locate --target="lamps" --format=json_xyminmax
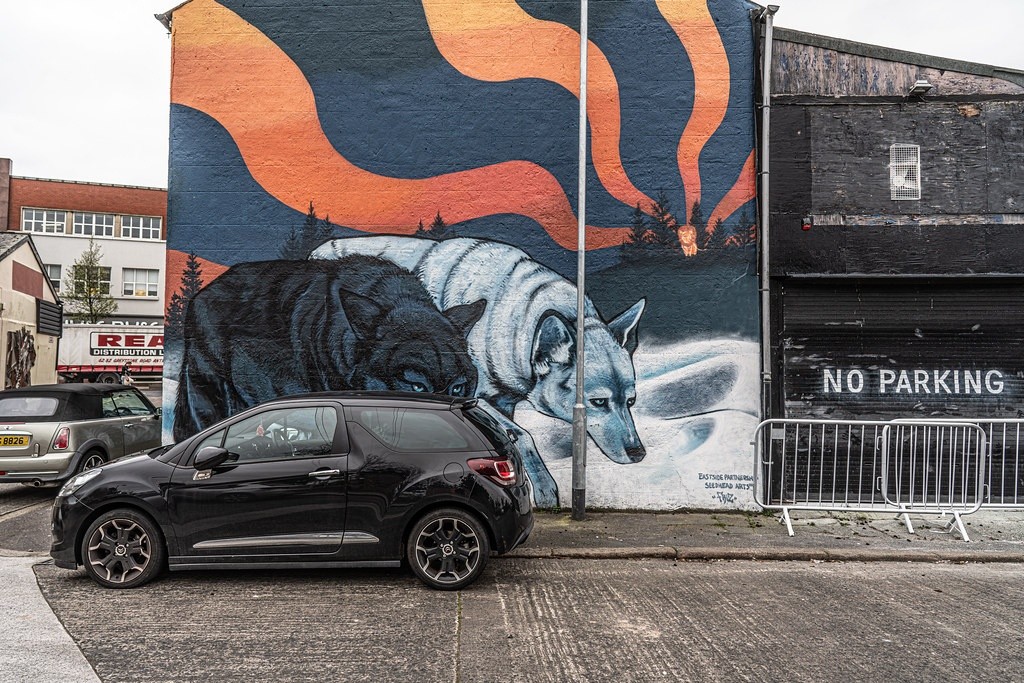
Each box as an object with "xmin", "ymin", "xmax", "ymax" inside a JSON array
[{"xmin": 909, "ymin": 79, "xmax": 935, "ymax": 95}]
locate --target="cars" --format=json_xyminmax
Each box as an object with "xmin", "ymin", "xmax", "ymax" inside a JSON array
[{"xmin": 0, "ymin": 382, "xmax": 163, "ymax": 500}]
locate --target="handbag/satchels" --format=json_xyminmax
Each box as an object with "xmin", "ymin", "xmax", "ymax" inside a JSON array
[{"xmin": 121, "ymin": 373, "xmax": 133, "ymax": 386}]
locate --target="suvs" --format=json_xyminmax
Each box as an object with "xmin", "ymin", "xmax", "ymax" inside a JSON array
[{"xmin": 49, "ymin": 389, "xmax": 539, "ymax": 590}]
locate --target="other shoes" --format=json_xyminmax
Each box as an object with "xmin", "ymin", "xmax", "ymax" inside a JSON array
[
  {"xmin": 118, "ymin": 395, "xmax": 123, "ymax": 397},
  {"xmin": 126, "ymin": 394, "xmax": 130, "ymax": 396}
]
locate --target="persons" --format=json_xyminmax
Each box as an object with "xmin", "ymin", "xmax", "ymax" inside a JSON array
[{"xmin": 119, "ymin": 362, "xmax": 134, "ymax": 397}]
